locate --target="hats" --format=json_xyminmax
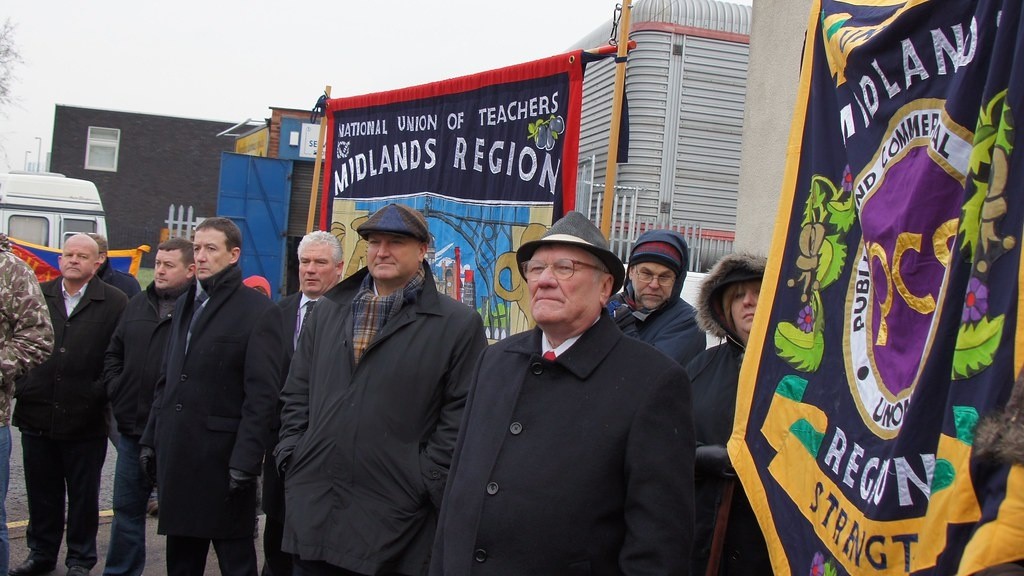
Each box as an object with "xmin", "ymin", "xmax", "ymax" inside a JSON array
[
  {"xmin": 629, "ymin": 241, "xmax": 683, "ymax": 277},
  {"xmin": 243, "ymin": 275, "xmax": 271, "ymax": 300},
  {"xmin": 516, "ymin": 211, "xmax": 625, "ymax": 298},
  {"xmin": 357, "ymin": 202, "xmax": 431, "ymax": 244}
]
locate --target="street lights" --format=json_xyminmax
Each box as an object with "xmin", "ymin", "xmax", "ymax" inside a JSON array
[
  {"xmin": 25, "ymin": 151, "xmax": 32, "ymax": 170},
  {"xmin": 35, "ymin": 138, "xmax": 41, "ymax": 173}
]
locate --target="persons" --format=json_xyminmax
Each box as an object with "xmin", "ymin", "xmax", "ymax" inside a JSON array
[
  {"xmin": 429, "ymin": 212, "xmax": 772, "ymax": 576},
  {"xmin": 0, "ymin": 217, "xmax": 344, "ymax": 576},
  {"xmin": 281, "ymin": 204, "xmax": 487, "ymax": 576},
  {"xmin": 940, "ymin": 365, "xmax": 1024, "ymax": 576}
]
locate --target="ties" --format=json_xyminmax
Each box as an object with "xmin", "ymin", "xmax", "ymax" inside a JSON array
[{"xmin": 297, "ymin": 300, "xmax": 315, "ymax": 337}]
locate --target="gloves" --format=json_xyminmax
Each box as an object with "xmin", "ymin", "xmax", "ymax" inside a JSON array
[
  {"xmin": 228, "ymin": 476, "xmax": 259, "ymax": 494},
  {"xmin": 696, "ymin": 442, "xmax": 739, "ymax": 478},
  {"xmin": 139, "ymin": 445, "xmax": 157, "ymax": 487}
]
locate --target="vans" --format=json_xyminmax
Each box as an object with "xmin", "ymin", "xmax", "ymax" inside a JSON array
[{"xmin": 0, "ymin": 171, "xmax": 109, "ymax": 251}]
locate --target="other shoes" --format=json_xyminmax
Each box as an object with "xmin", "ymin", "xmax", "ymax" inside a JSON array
[{"xmin": 147, "ymin": 492, "xmax": 159, "ymax": 515}]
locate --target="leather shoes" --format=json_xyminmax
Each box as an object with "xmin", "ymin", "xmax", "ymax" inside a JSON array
[
  {"xmin": 9, "ymin": 559, "xmax": 56, "ymax": 576},
  {"xmin": 66, "ymin": 566, "xmax": 89, "ymax": 576}
]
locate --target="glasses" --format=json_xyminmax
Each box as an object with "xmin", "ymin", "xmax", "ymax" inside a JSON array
[
  {"xmin": 634, "ymin": 264, "xmax": 675, "ymax": 286},
  {"xmin": 520, "ymin": 258, "xmax": 608, "ymax": 281}
]
